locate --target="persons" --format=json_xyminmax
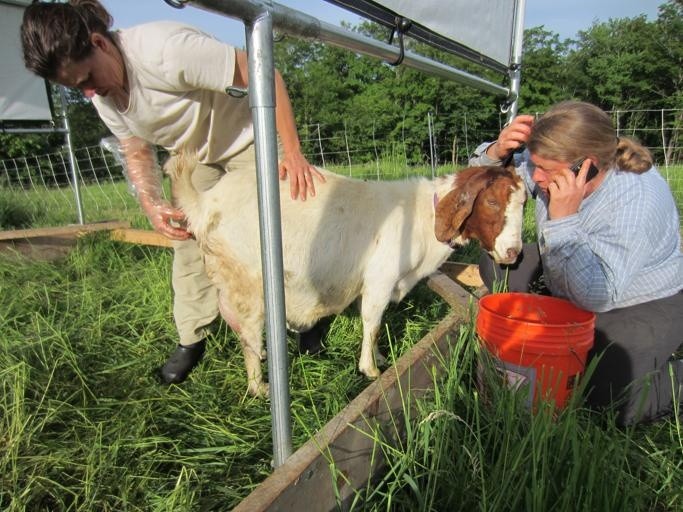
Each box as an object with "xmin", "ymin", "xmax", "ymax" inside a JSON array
[
  {"xmin": 21, "ymin": 0, "xmax": 326, "ymax": 381},
  {"xmin": 467, "ymin": 101, "xmax": 682, "ymax": 426}
]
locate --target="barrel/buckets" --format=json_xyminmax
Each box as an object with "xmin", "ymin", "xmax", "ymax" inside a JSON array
[{"xmin": 476, "ymin": 292, "xmax": 596, "ymax": 417}]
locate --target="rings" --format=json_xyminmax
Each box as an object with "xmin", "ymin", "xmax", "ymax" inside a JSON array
[{"xmin": 304, "ymin": 172, "xmax": 311, "ymax": 177}]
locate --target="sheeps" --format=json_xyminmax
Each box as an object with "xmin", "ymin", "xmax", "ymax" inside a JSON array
[{"xmin": 167, "ymin": 145, "xmax": 529, "ymax": 401}]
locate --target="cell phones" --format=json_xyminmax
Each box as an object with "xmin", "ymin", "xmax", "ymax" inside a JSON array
[{"xmin": 545, "ymin": 158, "xmax": 599, "ymax": 196}]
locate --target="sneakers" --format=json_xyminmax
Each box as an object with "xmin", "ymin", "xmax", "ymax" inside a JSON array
[
  {"xmin": 299, "ymin": 327, "xmax": 322, "ymax": 355},
  {"xmin": 162, "ymin": 345, "xmax": 202, "ymax": 382}
]
